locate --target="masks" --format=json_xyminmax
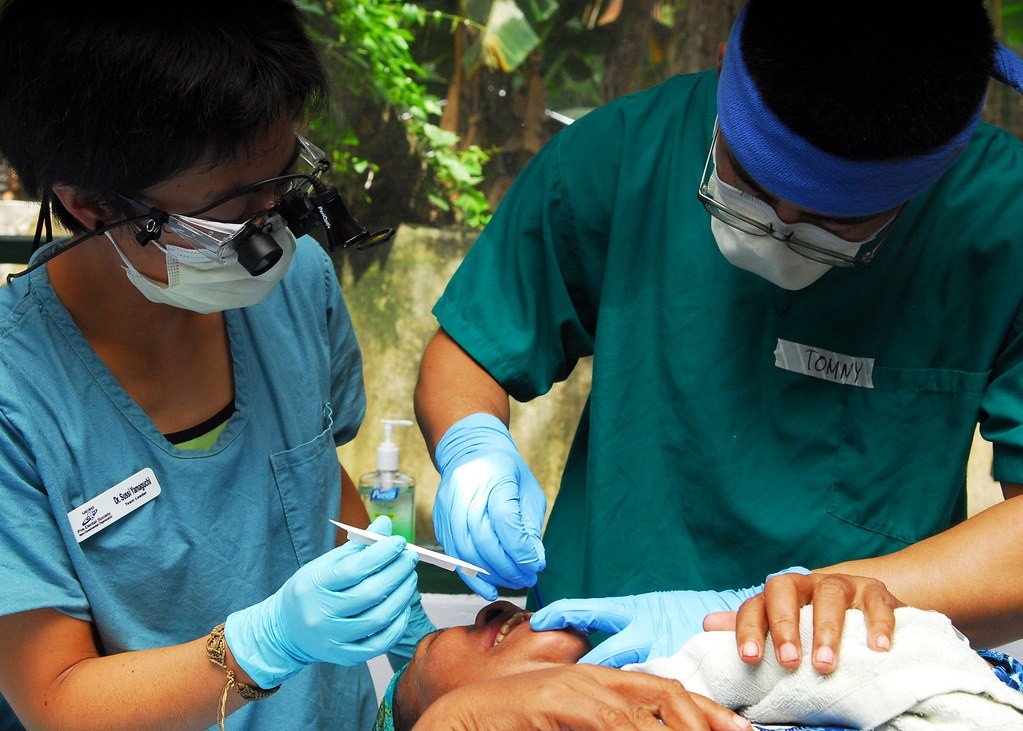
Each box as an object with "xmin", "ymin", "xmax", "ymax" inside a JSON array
[
  {"xmin": 95, "ymin": 201, "xmax": 298, "ymax": 315},
  {"xmin": 708, "ymin": 117, "xmax": 897, "ymax": 292}
]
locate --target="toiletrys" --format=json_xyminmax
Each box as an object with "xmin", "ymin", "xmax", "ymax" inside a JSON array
[{"xmin": 359, "ymin": 418, "xmax": 417, "ymax": 545}]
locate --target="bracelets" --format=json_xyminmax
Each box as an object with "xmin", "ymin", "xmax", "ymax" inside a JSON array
[{"xmin": 206, "ymin": 624, "xmax": 282, "ymax": 730}]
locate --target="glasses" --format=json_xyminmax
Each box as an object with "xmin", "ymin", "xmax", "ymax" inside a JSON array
[
  {"xmin": 118, "ymin": 129, "xmax": 334, "ymax": 264},
  {"xmin": 697, "ymin": 117, "xmax": 912, "ymax": 271}
]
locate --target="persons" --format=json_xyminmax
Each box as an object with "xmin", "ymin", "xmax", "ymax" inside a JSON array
[
  {"xmin": 373, "ymin": 601, "xmax": 1022, "ymax": 731},
  {"xmin": 0, "ymin": 0, "xmax": 435, "ymax": 731},
  {"xmin": 414, "ymin": 0, "xmax": 1022, "ymax": 673}
]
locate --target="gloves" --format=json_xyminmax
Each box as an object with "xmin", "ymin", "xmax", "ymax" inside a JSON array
[
  {"xmin": 433, "ymin": 414, "xmax": 548, "ymax": 600},
  {"xmin": 528, "ymin": 565, "xmax": 814, "ymax": 668},
  {"xmin": 220, "ymin": 514, "xmax": 417, "ymax": 688},
  {"xmin": 380, "ymin": 588, "xmax": 436, "ymax": 675}
]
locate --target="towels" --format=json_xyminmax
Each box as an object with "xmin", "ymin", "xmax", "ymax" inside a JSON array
[{"xmin": 620, "ymin": 605, "xmax": 1022, "ymax": 731}]
